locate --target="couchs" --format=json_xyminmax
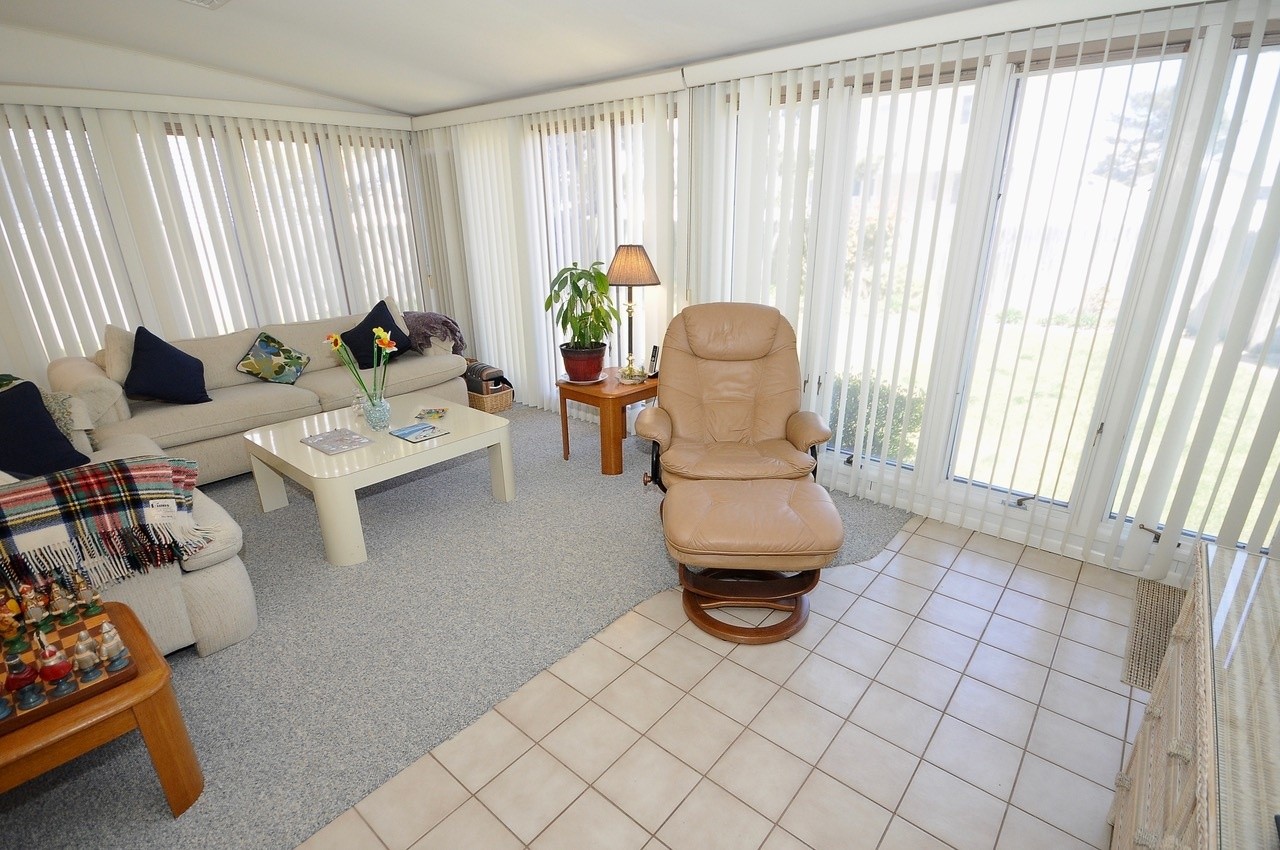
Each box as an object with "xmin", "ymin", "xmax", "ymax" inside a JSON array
[
  {"xmin": 44, "ymin": 312, "xmax": 467, "ymax": 489},
  {"xmin": 0, "ymin": 389, "xmax": 257, "ymax": 656}
]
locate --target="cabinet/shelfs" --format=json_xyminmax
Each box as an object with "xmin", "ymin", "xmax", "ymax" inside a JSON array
[{"xmin": 1112, "ymin": 543, "xmax": 1280, "ymax": 850}]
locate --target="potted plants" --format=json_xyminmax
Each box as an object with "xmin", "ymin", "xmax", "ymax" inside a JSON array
[{"xmin": 543, "ymin": 260, "xmax": 622, "ymax": 386}]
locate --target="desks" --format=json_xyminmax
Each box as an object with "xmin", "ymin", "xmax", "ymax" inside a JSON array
[
  {"xmin": 1, "ymin": 602, "xmax": 207, "ymax": 818},
  {"xmin": 556, "ymin": 367, "xmax": 659, "ymax": 475}
]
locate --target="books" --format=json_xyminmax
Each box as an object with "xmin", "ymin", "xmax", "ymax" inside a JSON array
[{"xmin": 300, "ymin": 427, "xmax": 377, "ymax": 456}]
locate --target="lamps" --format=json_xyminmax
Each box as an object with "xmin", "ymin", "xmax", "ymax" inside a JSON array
[{"xmin": 607, "ymin": 245, "xmax": 662, "ymax": 387}]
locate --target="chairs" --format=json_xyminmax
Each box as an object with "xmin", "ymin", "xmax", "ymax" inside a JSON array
[{"xmin": 635, "ymin": 304, "xmax": 844, "ymax": 647}]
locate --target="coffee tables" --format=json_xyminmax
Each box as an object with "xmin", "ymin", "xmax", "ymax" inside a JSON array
[{"xmin": 242, "ymin": 391, "xmax": 519, "ymax": 566}]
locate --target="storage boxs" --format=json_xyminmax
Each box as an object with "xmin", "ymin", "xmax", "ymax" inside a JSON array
[{"xmin": 463, "ymin": 362, "xmax": 504, "ymax": 394}]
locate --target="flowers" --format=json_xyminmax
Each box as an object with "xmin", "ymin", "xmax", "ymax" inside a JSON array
[{"xmin": 324, "ymin": 327, "xmax": 398, "ymax": 404}]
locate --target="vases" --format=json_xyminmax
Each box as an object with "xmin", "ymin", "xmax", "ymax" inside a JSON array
[{"xmin": 364, "ymin": 392, "xmax": 390, "ymax": 432}]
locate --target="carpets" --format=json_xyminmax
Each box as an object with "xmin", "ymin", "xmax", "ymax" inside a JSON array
[
  {"xmin": 0, "ymin": 399, "xmax": 921, "ymax": 849},
  {"xmin": 1123, "ymin": 578, "xmax": 1188, "ymax": 693}
]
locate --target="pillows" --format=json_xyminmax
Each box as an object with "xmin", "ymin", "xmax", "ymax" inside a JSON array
[
  {"xmin": 127, "ymin": 327, "xmax": 212, "ymax": 402},
  {"xmin": 101, "ymin": 323, "xmax": 135, "ymax": 384},
  {"xmin": 341, "ymin": 301, "xmax": 412, "ymax": 368},
  {"xmin": 385, "ymin": 296, "xmax": 410, "ymax": 336},
  {"xmin": 238, "ymin": 331, "xmax": 311, "ymax": 384}
]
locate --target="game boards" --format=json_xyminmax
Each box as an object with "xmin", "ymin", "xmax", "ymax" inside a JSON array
[{"xmin": 0, "ymin": 596, "xmax": 138, "ymax": 737}]
